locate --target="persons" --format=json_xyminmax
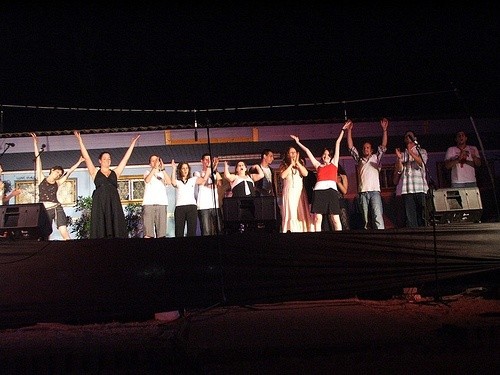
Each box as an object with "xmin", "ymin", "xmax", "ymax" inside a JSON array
[
  {"xmin": 74, "ymin": 130, "xmax": 140, "ymax": 239},
  {"xmin": 0, "ymin": 165, "xmax": 21, "ymax": 205},
  {"xmin": 444, "ymin": 130, "xmax": 481, "ymax": 188},
  {"xmin": 142, "ymin": 118, "xmax": 437, "ymax": 239},
  {"xmin": 29, "ymin": 133, "xmax": 85, "ymax": 240}
]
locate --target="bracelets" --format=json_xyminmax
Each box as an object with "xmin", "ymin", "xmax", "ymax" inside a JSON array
[
  {"xmin": 383, "ymin": 129, "xmax": 387, "ymax": 132},
  {"xmin": 341, "ymin": 129, "xmax": 345, "ymax": 131},
  {"xmin": 161, "ymin": 168, "xmax": 165, "ymax": 171},
  {"xmin": 457, "ymin": 158, "xmax": 460, "ymax": 162}
]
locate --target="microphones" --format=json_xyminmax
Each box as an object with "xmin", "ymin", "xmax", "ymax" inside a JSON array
[
  {"xmin": 194, "ymin": 120, "xmax": 198, "ymax": 141},
  {"xmin": 40, "ymin": 144, "xmax": 46, "ymax": 152},
  {"xmin": 408, "ymin": 135, "xmax": 421, "ymax": 149},
  {"xmin": 6, "ymin": 143, "xmax": 15, "ymax": 147}
]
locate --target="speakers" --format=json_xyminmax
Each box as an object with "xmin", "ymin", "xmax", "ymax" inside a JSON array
[
  {"xmin": 425, "ymin": 187, "xmax": 483, "ymax": 226},
  {"xmin": 222, "ymin": 195, "xmax": 282, "ymax": 234},
  {"xmin": 0, "ymin": 203, "xmax": 52, "ymax": 241}
]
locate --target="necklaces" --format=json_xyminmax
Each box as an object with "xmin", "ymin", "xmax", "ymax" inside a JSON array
[{"xmin": 291, "ymin": 167, "xmax": 297, "ymax": 175}]
